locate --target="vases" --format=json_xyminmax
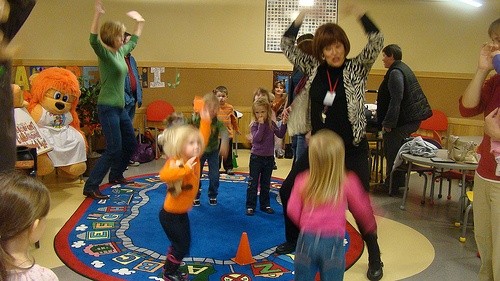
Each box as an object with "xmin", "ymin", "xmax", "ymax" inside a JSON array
[{"xmin": 85, "ymin": 151, "xmax": 102, "ymax": 177}]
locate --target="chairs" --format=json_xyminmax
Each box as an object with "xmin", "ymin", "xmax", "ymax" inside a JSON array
[
  {"xmin": 136, "ymin": 100, "xmax": 175, "ymax": 160},
  {"xmin": 388, "ymin": 110, "xmax": 475, "ymax": 242}
]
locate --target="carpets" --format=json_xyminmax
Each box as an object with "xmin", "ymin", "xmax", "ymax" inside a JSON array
[{"xmin": 54, "ymin": 169, "xmax": 365, "ymax": 281}]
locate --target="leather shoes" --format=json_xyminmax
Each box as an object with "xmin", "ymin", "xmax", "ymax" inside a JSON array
[
  {"xmin": 84, "ymin": 189, "xmax": 108, "ymax": 199},
  {"xmin": 276, "ymin": 242, "xmax": 296, "ymax": 254},
  {"xmin": 109, "ymin": 177, "xmax": 134, "ymax": 184},
  {"xmin": 363, "ymin": 259, "xmax": 383, "ymax": 281}
]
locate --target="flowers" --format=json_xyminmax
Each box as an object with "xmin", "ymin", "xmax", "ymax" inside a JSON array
[{"xmin": 75, "ymin": 76, "xmax": 103, "ymax": 138}]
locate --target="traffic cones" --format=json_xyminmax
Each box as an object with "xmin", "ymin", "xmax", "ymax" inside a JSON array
[{"xmin": 230, "ymin": 232, "xmax": 257, "ymax": 265}]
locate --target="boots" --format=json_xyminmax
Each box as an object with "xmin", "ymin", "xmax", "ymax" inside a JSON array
[
  {"xmin": 162, "ymin": 246, "xmax": 173, "ymax": 272},
  {"xmin": 163, "ymin": 253, "xmax": 189, "ymax": 281}
]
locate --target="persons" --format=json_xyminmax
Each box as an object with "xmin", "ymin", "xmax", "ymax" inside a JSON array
[
  {"xmin": 122, "ymin": 31, "xmax": 140, "ymax": 169},
  {"xmin": 159, "ymin": 109, "xmax": 211, "ymax": 281},
  {"xmin": 246, "ymin": 80, "xmax": 288, "ymax": 215},
  {"xmin": 376, "ymin": 43, "xmax": 433, "ymax": 193},
  {"xmin": 82, "ymin": 0, "xmax": 145, "ymax": 199},
  {"xmin": 184, "ymin": 86, "xmax": 240, "ymax": 208},
  {"xmin": 459, "ymin": 16, "xmax": 500, "ymax": 281},
  {"xmin": 286, "ymin": 129, "xmax": 376, "ymax": 281},
  {"xmin": 276, "ymin": 9, "xmax": 385, "ymax": 281},
  {"xmin": 289, "ymin": 31, "xmax": 317, "ymax": 162},
  {"xmin": 0, "ymin": 170, "xmax": 59, "ymax": 281}
]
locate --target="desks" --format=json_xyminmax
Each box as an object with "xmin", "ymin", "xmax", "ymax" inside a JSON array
[{"xmin": 400, "ymin": 136, "xmax": 484, "ymax": 225}]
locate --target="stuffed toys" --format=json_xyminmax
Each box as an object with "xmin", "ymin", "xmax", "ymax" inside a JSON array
[{"xmin": 23, "ymin": 65, "xmax": 88, "ymax": 176}]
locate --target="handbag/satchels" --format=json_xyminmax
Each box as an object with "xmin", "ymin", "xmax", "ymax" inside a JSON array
[
  {"xmin": 284, "ymin": 143, "xmax": 294, "ymax": 159},
  {"xmin": 132, "ymin": 131, "xmax": 160, "ymax": 163},
  {"xmin": 448, "ymin": 135, "xmax": 479, "ymax": 165}
]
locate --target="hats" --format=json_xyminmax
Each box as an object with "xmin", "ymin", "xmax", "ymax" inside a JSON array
[{"xmin": 297, "ymin": 33, "xmax": 314, "ymax": 46}]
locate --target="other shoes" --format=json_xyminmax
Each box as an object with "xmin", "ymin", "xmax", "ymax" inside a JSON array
[
  {"xmin": 209, "ymin": 198, "xmax": 217, "ymax": 205},
  {"xmin": 192, "ymin": 200, "xmax": 200, "ymax": 206},
  {"xmin": 374, "ymin": 184, "xmax": 398, "ymax": 194},
  {"xmin": 247, "ymin": 208, "xmax": 254, "ymax": 215},
  {"xmin": 227, "ymin": 169, "xmax": 235, "ymax": 174},
  {"xmin": 264, "ymin": 207, "xmax": 273, "ymax": 214}
]
